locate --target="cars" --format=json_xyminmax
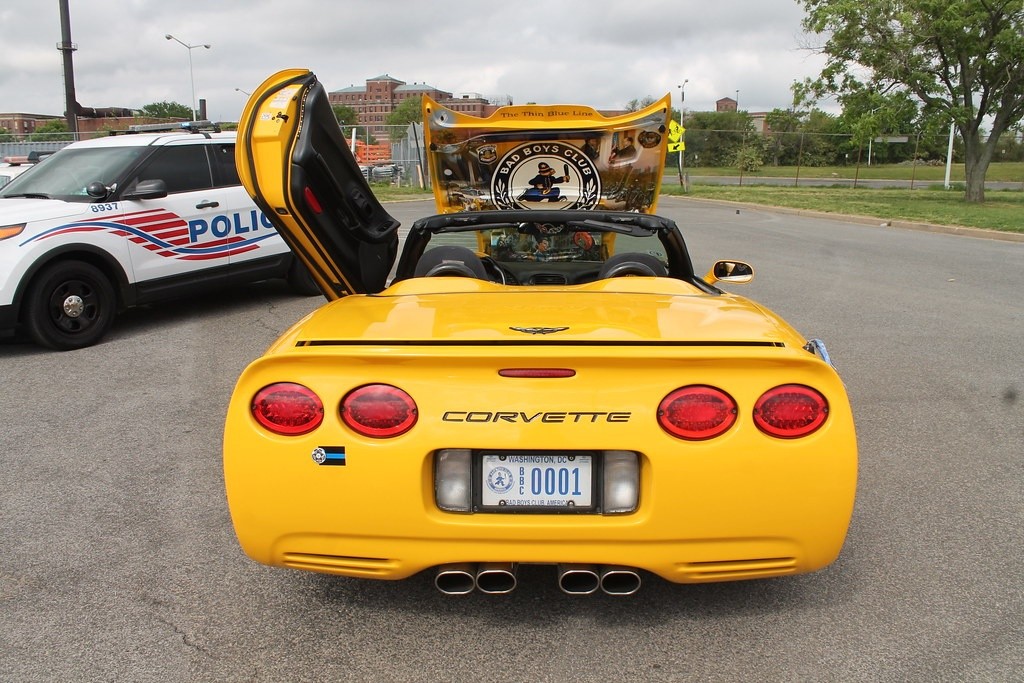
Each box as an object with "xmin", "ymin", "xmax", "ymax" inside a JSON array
[{"xmin": 0, "ymin": 120, "xmax": 325, "ymax": 351}]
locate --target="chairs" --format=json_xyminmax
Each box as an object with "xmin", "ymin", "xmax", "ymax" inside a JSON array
[
  {"xmin": 596, "ymin": 252, "xmax": 668, "ymax": 281},
  {"xmin": 413, "ymin": 245, "xmax": 489, "ymax": 282}
]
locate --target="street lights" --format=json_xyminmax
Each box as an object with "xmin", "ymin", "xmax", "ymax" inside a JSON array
[
  {"xmin": 165, "ymin": 33, "xmax": 211, "ymax": 120},
  {"xmin": 677, "ymin": 78, "xmax": 689, "ymax": 177}
]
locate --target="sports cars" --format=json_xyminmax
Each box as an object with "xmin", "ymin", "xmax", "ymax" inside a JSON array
[{"xmin": 222, "ymin": 68, "xmax": 861, "ymax": 596}]
